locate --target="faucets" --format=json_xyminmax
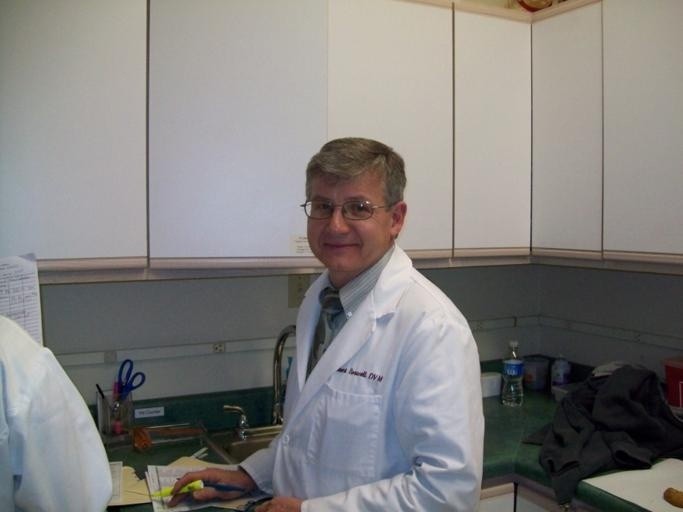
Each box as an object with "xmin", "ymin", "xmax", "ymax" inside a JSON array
[{"xmin": 271, "ymin": 325, "xmax": 296, "ymax": 426}]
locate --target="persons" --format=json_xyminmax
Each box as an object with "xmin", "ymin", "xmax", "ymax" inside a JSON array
[
  {"xmin": 1, "ymin": 313, "xmax": 115, "ymax": 512},
  {"xmin": 166, "ymin": 137, "xmax": 486, "ymax": 512}
]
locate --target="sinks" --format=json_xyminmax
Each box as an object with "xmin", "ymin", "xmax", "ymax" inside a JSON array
[{"xmin": 195, "ymin": 425, "xmax": 283, "ymax": 465}]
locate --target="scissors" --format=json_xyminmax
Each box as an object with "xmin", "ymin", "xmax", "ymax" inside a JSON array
[{"xmin": 111, "ymin": 359, "xmax": 145, "ymax": 414}]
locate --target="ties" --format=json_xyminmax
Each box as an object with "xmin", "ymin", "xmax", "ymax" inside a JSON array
[{"xmin": 306, "ymin": 287, "xmax": 342, "ymax": 386}]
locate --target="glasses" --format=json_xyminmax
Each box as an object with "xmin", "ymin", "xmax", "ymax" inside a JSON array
[{"xmin": 300, "ymin": 199, "xmax": 393, "ymax": 220}]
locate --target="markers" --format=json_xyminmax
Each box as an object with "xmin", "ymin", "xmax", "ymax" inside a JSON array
[{"xmin": 149, "ymin": 480, "xmax": 204, "ymax": 498}]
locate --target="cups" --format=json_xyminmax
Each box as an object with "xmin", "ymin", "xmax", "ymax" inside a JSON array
[
  {"xmin": 525, "ymin": 357, "xmax": 550, "ymax": 390},
  {"xmin": 94, "ymin": 389, "xmax": 134, "ymax": 444}
]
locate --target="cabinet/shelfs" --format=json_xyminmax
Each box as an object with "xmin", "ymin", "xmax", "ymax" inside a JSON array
[
  {"xmin": 476, "ymin": 480, "xmax": 516, "ymax": 511},
  {"xmin": 518, "ymin": 485, "xmax": 580, "ymax": 512},
  {"xmin": 533, "ymin": 1, "xmax": 683, "ymax": 275},
  {"xmin": 328, "ymin": 1, "xmax": 533, "ymax": 269},
  {"xmin": 1, "ymin": 2, "xmax": 326, "ymax": 273}
]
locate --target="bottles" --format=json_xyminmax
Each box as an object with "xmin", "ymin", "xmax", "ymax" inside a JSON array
[{"xmin": 500, "ymin": 340, "xmax": 525, "ymax": 408}]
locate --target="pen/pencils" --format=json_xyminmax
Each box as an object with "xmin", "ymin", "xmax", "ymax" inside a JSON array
[
  {"xmin": 96, "ymin": 382, "xmax": 123, "ymax": 435},
  {"xmin": 176, "ymin": 478, "xmax": 247, "ymax": 492}
]
